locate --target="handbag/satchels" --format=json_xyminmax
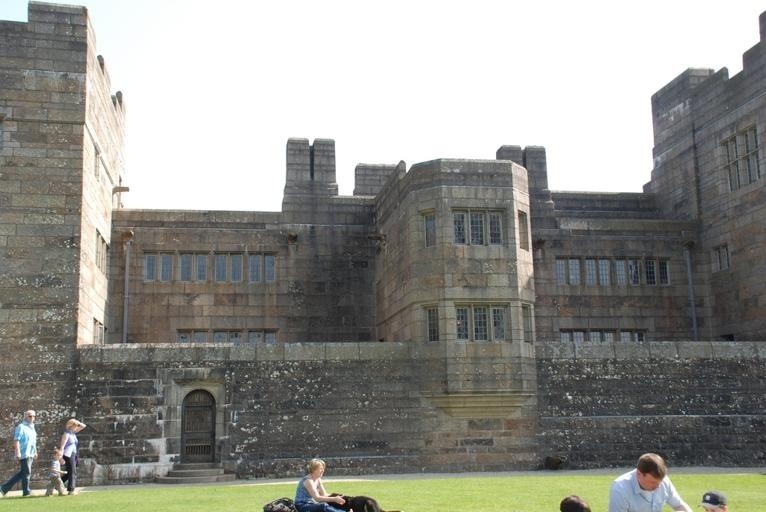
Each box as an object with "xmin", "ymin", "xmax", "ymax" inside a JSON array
[
  {"xmin": 75, "ymin": 454, "xmax": 79, "ymax": 465},
  {"xmin": 264, "ymin": 497, "xmax": 295, "ymax": 512}
]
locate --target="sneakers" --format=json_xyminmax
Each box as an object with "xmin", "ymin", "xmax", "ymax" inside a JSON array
[
  {"xmin": 0, "ymin": 485, "xmax": 6, "ymax": 496},
  {"xmin": 22, "ymin": 492, "xmax": 78, "ymax": 497}
]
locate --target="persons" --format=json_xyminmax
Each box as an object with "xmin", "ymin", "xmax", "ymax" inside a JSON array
[
  {"xmin": 558, "ymin": 495, "xmax": 593, "ymax": 512},
  {"xmin": 0, "ymin": 409, "xmax": 38, "ymax": 499},
  {"xmin": 294, "ymin": 458, "xmax": 347, "ymax": 512},
  {"xmin": 52, "ymin": 417, "xmax": 86, "ymax": 495},
  {"xmin": 44, "ymin": 448, "xmax": 71, "ymax": 496},
  {"xmin": 696, "ymin": 490, "xmax": 728, "ymax": 512},
  {"xmin": 607, "ymin": 452, "xmax": 693, "ymax": 512}
]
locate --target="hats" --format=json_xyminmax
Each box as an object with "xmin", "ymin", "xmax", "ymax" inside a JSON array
[
  {"xmin": 66, "ymin": 419, "xmax": 87, "ymax": 431},
  {"xmin": 697, "ymin": 492, "xmax": 725, "ymax": 510}
]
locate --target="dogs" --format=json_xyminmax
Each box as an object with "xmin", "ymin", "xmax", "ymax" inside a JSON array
[{"xmin": 328, "ymin": 493, "xmax": 401, "ymax": 512}]
